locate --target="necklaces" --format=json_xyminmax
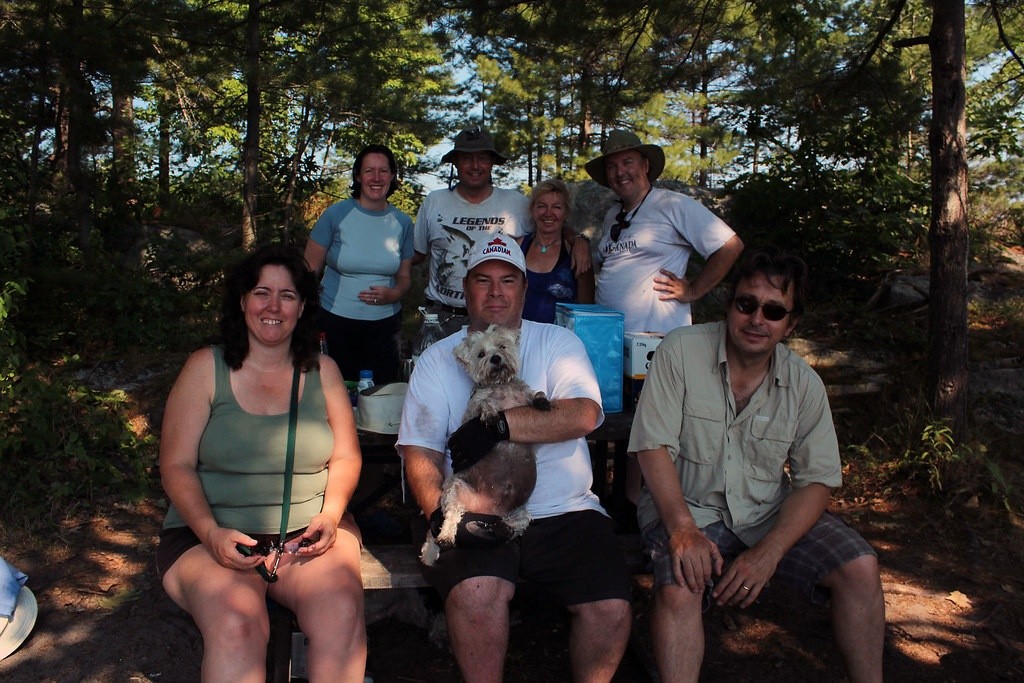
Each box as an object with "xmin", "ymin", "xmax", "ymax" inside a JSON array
[
  {"xmin": 616, "ymin": 183, "xmax": 653, "ymax": 225},
  {"xmin": 534, "ymin": 235, "xmax": 560, "ymax": 253}
]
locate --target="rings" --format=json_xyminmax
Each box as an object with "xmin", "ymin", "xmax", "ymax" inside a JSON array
[
  {"xmin": 743, "ymin": 585, "xmax": 750, "ymax": 591},
  {"xmin": 373, "ymin": 298, "xmax": 377, "ymax": 304}
]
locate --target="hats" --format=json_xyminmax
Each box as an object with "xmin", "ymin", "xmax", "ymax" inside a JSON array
[
  {"xmin": 585, "ymin": 130, "xmax": 665, "ymax": 188},
  {"xmin": 467, "ymin": 233, "xmax": 526, "ymax": 281},
  {"xmin": 0, "ymin": 586, "xmax": 38, "ymax": 661},
  {"xmin": 441, "ymin": 130, "xmax": 507, "ymax": 166}
]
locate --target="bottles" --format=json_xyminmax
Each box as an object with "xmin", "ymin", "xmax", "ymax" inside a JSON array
[
  {"xmin": 319, "ymin": 333, "xmax": 328, "ymax": 354},
  {"xmin": 411, "ymin": 306, "xmax": 447, "ymax": 366},
  {"xmin": 357, "ymin": 369, "xmax": 374, "ymax": 394}
]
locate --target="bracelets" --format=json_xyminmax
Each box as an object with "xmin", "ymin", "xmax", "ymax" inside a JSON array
[{"xmin": 575, "ymin": 234, "xmax": 590, "ymax": 241}]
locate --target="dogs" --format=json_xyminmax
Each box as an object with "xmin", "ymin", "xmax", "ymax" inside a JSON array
[{"xmin": 420, "ymin": 327, "xmax": 550, "ymax": 567}]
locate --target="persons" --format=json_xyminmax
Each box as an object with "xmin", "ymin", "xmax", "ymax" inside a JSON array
[
  {"xmin": 584, "ymin": 130, "xmax": 744, "ymax": 333},
  {"xmin": 618, "ymin": 247, "xmax": 887, "ymax": 683},
  {"xmin": 302, "ymin": 144, "xmax": 414, "ymax": 385},
  {"xmin": 152, "ymin": 238, "xmax": 369, "ymax": 683},
  {"xmin": 411, "ymin": 128, "xmax": 592, "ymax": 355},
  {"xmin": 512, "ymin": 180, "xmax": 596, "ymax": 324},
  {"xmin": 397, "ymin": 234, "xmax": 634, "ymax": 683}
]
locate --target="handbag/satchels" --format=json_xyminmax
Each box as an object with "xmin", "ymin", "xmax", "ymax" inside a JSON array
[{"xmin": 554, "ymin": 301, "xmax": 624, "ymax": 414}]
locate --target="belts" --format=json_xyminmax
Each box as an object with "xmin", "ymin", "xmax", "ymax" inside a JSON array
[{"xmin": 424, "ymin": 296, "xmax": 468, "ymax": 316}]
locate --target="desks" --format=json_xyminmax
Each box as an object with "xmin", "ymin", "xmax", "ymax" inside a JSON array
[{"xmin": 358, "ymin": 396, "xmax": 855, "ymax": 533}]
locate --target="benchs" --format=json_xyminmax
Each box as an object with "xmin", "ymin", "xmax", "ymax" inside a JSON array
[{"xmin": 265, "ymin": 534, "xmax": 776, "ymax": 683}]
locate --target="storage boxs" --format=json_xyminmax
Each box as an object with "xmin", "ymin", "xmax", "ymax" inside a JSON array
[{"xmin": 624, "ymin": 332, "xmax": 666, "ymax": 410}]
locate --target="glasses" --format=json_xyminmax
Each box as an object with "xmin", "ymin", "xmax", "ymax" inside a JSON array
[{"xmin": 729, "ymin": 293, "xmax": 793, "ymax": 321}]
